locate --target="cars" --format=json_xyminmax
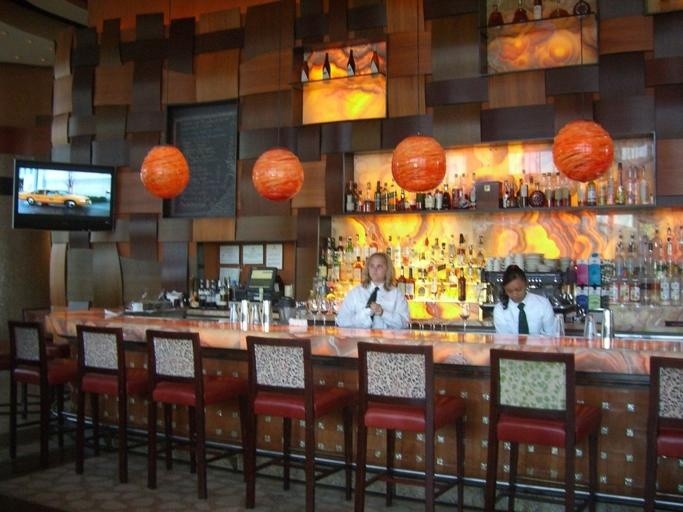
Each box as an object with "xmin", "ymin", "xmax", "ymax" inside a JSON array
[{"xmin": 18, "ymin": 188, "xmax": 92, "ymax": 211}]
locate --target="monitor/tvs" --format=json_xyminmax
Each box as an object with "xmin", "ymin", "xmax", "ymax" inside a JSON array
[
  {"xmin": 11, "ymin": 158, "xmax": 116, "ymax": 232},
  {"xmin": 246, "ymin": 267, "xmax": 277, "ymax": 292}
]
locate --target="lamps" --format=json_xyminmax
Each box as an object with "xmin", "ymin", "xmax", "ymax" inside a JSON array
[
  {"xmin": 252, "ymin": 0, "xmax": 303, "ymax": 202},
  {"xmin": 554, "ymin": 0, "xmax": 614, "ymax": 182},
  {"xmin": 141, "ymin": 1, "xmax": 190, "ymax": 199},
  {"xmin": 391, "ymin": 0, "xmax": 447, "ymax": 192}
]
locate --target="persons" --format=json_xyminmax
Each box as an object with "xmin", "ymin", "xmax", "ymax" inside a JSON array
[
  {"xmin": 334, "ymin": 251, "xmax": 412, "ymax": 329},
  {"xmin": 492, "ymin": 264, "xmax": 560, "ymax": 337}
]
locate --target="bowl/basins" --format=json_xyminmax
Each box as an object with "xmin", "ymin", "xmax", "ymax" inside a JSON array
[{"xmin": 525, "ymin": 254, "xmax": 571, "ymax": 271}]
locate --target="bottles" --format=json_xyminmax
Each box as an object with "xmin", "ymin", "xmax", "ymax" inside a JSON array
[
  {"xmin": 610, "ymin": 226, "xmax": 682, "ymax": 305},
  {"xmin": 229, "ymin": 299, "xmax": 273, "ymax": 331},
  {"xmin": 198, "ymin": 277, "xmax": 233, "ymax": 308},
  {"xmin": 369, "ymin": 304, "xmax": 384, "ymax": 337},
  {"xmin": 315, "ymin": 235, "xmax": 481, "ymax": 298},
  {"xmin": 344, "ymin": 161, "xmax": 648, "ymax": 214}
]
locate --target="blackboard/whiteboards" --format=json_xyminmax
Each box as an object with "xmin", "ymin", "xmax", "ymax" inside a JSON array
[{"xmin": 171, "ymin": 112, "xmax": 237, "ymax": 216}]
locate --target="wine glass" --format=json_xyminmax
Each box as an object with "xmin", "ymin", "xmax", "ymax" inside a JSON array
[
  {"xmin": 310, "ymin": 299, "xmax": 339, "ymax": 327},
  {"xmin": 403, "ymin": 315, "xmax": 450, "ymax": 339},
  {"xmin": 458, "ymin": 303, "xmax": 470, "ymax": 330}
]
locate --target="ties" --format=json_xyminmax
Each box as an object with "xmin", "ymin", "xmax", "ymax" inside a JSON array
[
  {"xmin": 366, "ymin": 286, "xmax": 379, "ymax": 319},
  {"xmin": 518, "ymin": 303, "xmax": 529, "ymax": 334}
]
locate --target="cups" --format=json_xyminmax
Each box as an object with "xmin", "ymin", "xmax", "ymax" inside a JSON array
[{"xmin": 485, "ymin": 252, "xmax": 523, "ymax": 271}]
